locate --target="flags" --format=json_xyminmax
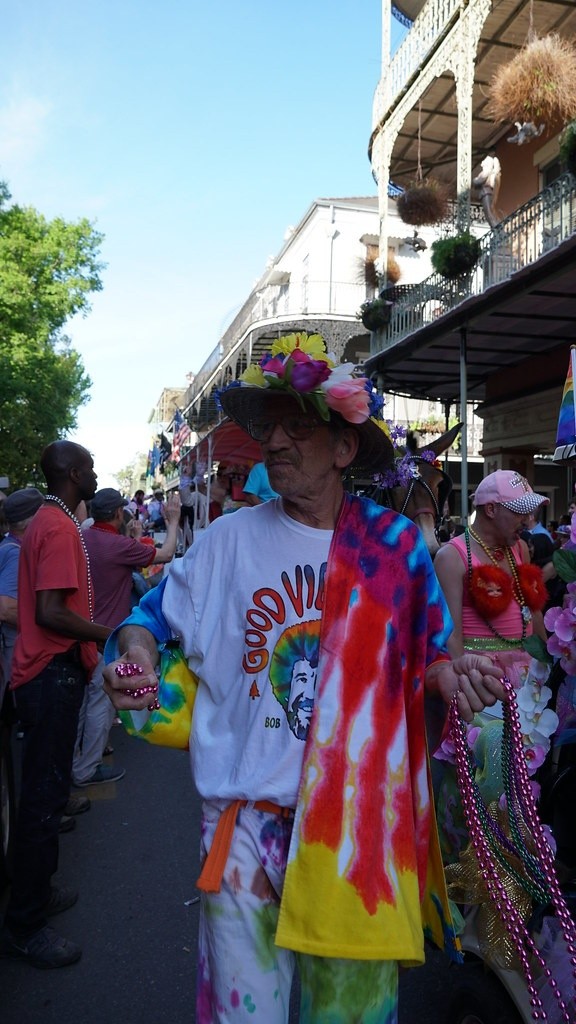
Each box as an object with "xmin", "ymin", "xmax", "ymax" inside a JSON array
[
  {"xmin": 146, "ymin": 431, "xmax": 172, "ymax": 477},
  {"xmin": 174, "ymin": 409, "xmax": 192, "ymax": 448}
]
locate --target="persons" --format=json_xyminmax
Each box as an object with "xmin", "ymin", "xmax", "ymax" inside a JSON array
[
  {"xmin": 474, "ymin": 145, "xmax": 509, "ymax": 246},
  {"xmin": 68, "ymin": 488, "xmax": 181, "ymax": 789},
  {"xmin": 101, "ymin": 335, "xmax": 506, "ymax": 1024},
  {"xmin": 436, "ymin": 470, "xmax": 556, "ymax": 855},
  {"xmin": 521, "ymin": 501, "xmax": 576, "ymax": 920},
  {"xmin": 174, "ymin": 456, "xmax": 280, "ymax": 555},
  {"xmin": 0, "ymin": 442, "xmax": 114, "ymax": 968},
  {"xmin": 0, "ymin": 488, "xmax": 91, "ymax": 832}
]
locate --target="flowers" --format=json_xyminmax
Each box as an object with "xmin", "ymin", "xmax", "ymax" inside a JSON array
[{"xmin": 219, "ymin": 333, "xmax": 391, "ymax": 424}]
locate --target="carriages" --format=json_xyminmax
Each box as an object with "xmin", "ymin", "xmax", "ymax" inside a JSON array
[{"xmin": 172, "ymin": 313, "xmax": 465, "ymax": 563}]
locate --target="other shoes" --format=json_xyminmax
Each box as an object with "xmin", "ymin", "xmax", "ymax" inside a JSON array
[
  {"xmin": 17, "ymin": 724, "xmax": 25, "ymax": 738},
  {"xmin": 102, "ymin": 747, "xmax": 114, "ymax": 756}
]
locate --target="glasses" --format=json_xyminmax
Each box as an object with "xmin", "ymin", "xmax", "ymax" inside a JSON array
[{"xmin": 245, "ymin": 414, "xmax": 339, "ymax": 441}]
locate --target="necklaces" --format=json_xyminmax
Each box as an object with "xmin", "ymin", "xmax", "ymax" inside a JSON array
[
  {"xmin": 8, "ymin": 533, "xmax": 22, "ymax": 543},
  {"xmin": 451, "ymin": 679, "xmax": 575, "ymax": 1024},
  {"xmin": 45, "ymin": 495, "xmax": 94, "ymax": 622},
  {"xmin": 115, "ymin": 663, "xmax": 161, "ymax": 712},
  {"xmin": 464, "ymin": 526, "xmax": 527, "ymax": 644}
]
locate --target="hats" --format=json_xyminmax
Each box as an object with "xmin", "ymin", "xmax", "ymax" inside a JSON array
[
  {"xmin": 215, "ymin": 332, "xmax": 394, "ymax": 477},
  {"xmin": 153, "ymin": 489, "xmax": 163, "ymax": 496},
  {"xmin": 90, "ymin": 488, "xmax": 129, "ymax": 518},
  {"xmin": 3, "ymin": 488, "xmax": 44, "ymax": 522},
  {"xmin": 555, "ymin": 525, "xmax": 572, "ymax": 535},
  {"xmin": 470, "ymin": 469, "xmax": 550, "ymax": 514}
]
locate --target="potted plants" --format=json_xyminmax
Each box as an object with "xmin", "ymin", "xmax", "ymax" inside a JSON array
[
  {"xmin": 432, "ymin": 230, "xmax": 481, "ymax": 279},
  {"xmin": 356, "ymin": 297, "xmax": 394, "ymax": 332}
]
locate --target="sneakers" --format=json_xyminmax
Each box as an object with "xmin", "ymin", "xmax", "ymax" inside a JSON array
[
  {"xmin": 75, "ymin": 763, "xmax": 125, "ymax": 787},
  {"xmin": 64, "ymin": 796, "xmax": 90, "ymax": 815},
  {"xmin": 57, "ymin": 815, "xmax": 75, "ymax": 834},
  {"xmin": 0, "ymin": 923, "xmax": 83, "ymax": 968},
  {"xmin": 46, "ymin": 887, "xmax": 78, "ymax": 918}
]
